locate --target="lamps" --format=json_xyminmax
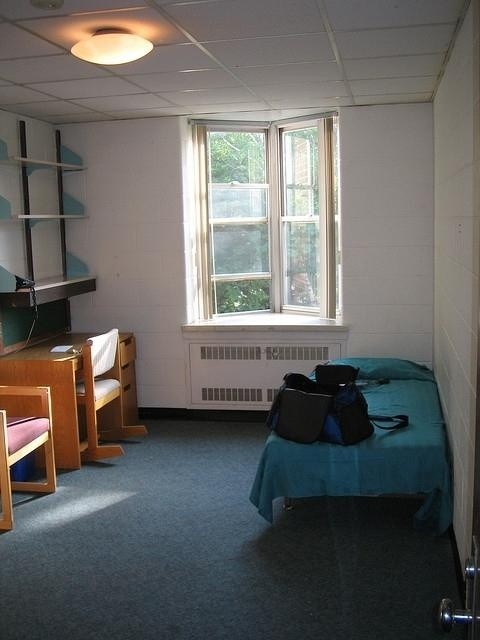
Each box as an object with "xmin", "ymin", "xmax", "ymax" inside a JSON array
[{"xmin": 71, "ymin": 28, "xmax": 155, "ymax": 66}]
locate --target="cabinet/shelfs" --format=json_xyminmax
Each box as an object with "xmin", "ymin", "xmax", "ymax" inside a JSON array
[{"xmin": 1, "ymin": 120, "xmax": 97, "ymax": 310}]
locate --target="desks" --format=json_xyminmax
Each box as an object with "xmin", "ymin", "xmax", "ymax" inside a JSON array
[{"xmin": 1, "ymin": 333, "xmax": 140, "ymax": 469}]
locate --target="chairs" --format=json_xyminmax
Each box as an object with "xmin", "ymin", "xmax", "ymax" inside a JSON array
[
  {"xmin": 0, "ymin": 385, "xmax": 57, "ymax": 530},
  {"xmin": 76, "ymin": 329, "xmax": 148, "ymax": 463}
]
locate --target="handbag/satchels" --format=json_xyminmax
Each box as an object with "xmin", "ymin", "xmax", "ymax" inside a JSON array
[{"xmin": 266, "ymin": 365, "xmax": 375, "ymax": 447}]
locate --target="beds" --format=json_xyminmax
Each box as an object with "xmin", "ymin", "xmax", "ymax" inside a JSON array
[{"xmin": 248, "ymin": 379, "xmax": 453, "ymax": 538}]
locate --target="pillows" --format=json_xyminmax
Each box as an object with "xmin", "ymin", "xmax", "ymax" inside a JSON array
[{"xmin": 308, "ymin": 356, "xmax": 434, "ymax": 382}]
